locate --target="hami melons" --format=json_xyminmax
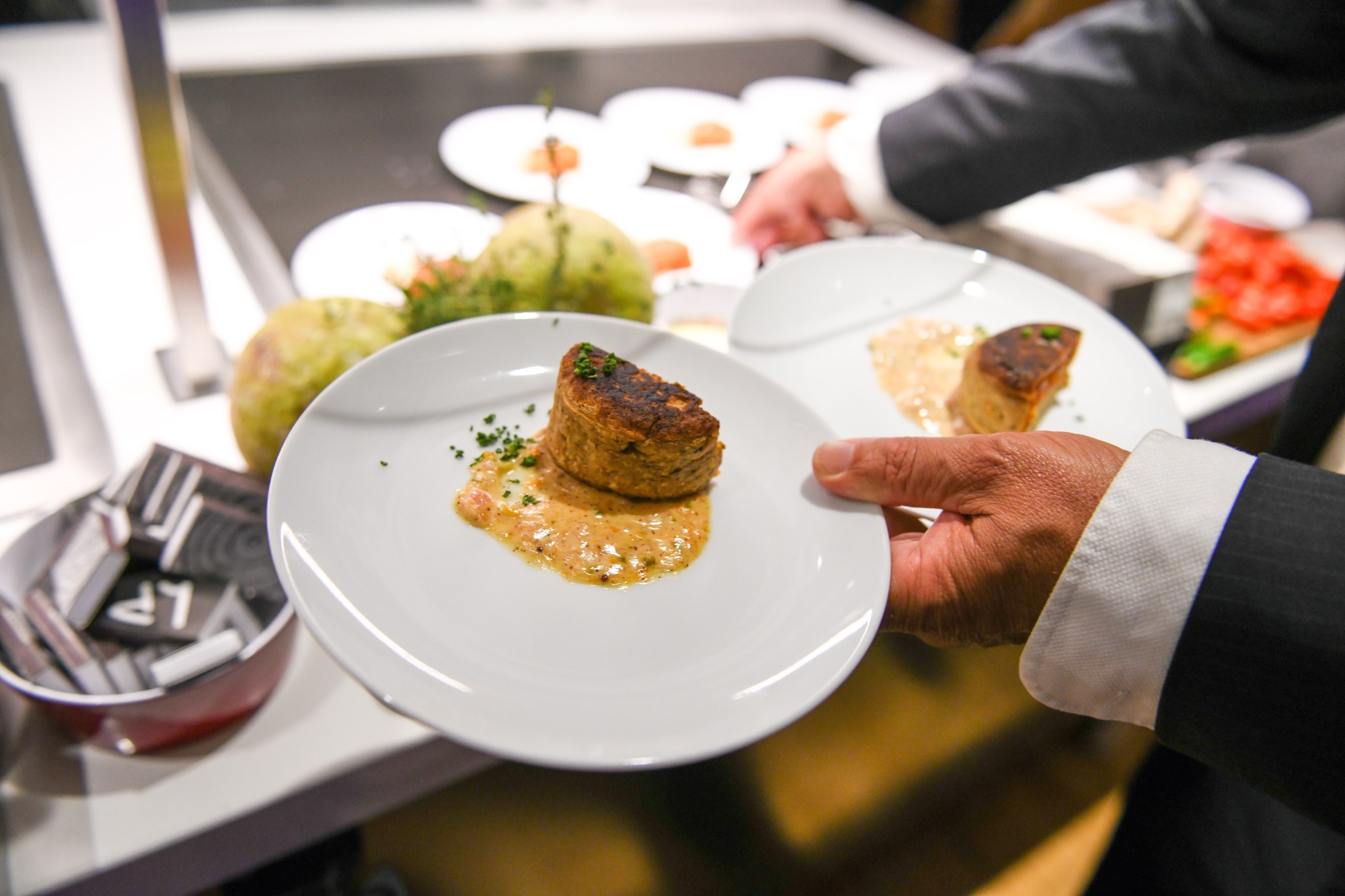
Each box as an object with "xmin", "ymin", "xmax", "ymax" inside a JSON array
[{"xmin": 231, "ymin": 204, "xmax": 654, "ymax": 479}]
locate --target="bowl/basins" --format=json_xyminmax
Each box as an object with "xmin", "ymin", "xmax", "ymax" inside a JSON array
[
  {"xmin": 0, "ymin": 485, "xmax": 300, "ymax": 757},
  {"xmin": 1183, "ymin": 161, "xmax": 1308, "ymax": 284}
]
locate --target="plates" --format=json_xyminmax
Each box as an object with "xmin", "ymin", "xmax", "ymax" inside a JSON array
[
  {"xmin": 725, "ymin": 237, "xmax": 1186, "ymax": 521},
  {"xmin": 289, "ymin": 66, "xmax": 974, "ymax": 326},
  {"xmin": 267, "ymin": 309, "xmax": 893, "ymax": 773}
]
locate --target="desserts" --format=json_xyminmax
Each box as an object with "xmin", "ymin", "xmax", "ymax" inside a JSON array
[{"xmin": 406, "ymin": 113, "xmax": 853, "ymax": 299}]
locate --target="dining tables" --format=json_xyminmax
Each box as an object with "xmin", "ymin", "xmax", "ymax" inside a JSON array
[{"xmin": 0, "ymin": 0, "xmax": 1345, "ymax": 896}]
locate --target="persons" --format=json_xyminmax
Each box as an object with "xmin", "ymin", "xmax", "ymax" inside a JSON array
[{"xmin": 728, "ymin": 0, "xmax": 1345, "ymax": 894}]
